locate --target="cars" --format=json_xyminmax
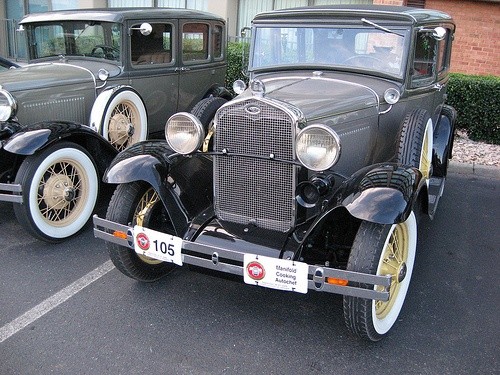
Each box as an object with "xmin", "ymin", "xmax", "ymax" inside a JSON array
[
  {"xmin": 0, "ymin": 7, "xmax": 233, "ymax": 244},
  {"xmin": 93, "ymin": 4, "xmax": 457, "ymax": 342}
]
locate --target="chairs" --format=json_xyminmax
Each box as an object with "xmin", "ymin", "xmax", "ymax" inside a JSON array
[{"xmin": 136, "ymin": 53, "xmax": 172, "ymax": 65}]
